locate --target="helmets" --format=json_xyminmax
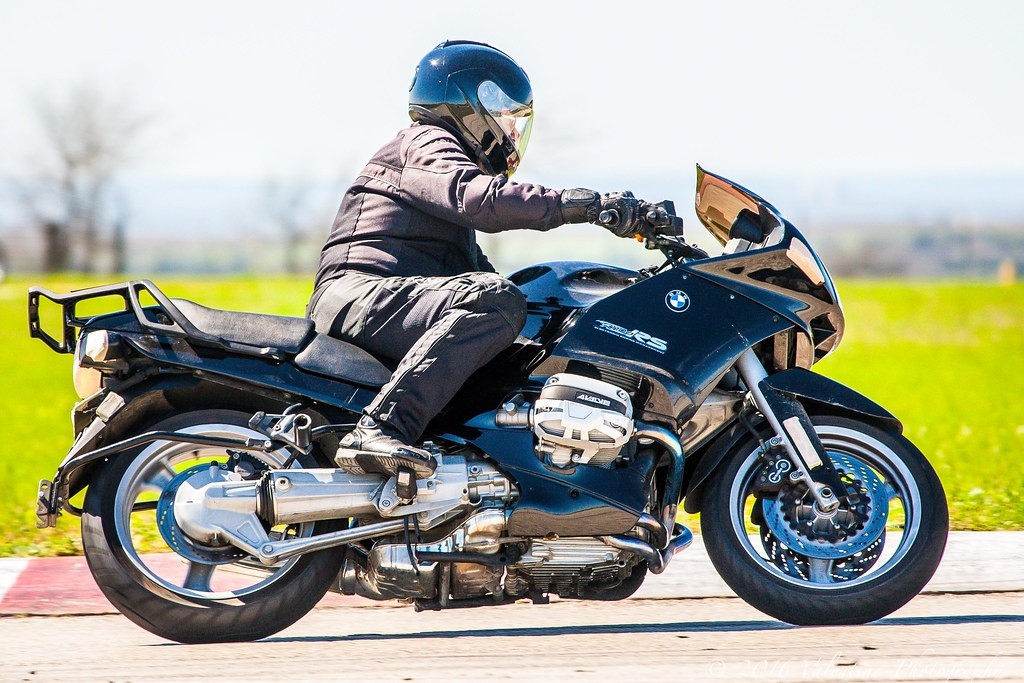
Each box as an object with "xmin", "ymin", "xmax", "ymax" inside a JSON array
[{"xmin": 408, "ymin": 36, "xmax": 534, "ymax": 181}]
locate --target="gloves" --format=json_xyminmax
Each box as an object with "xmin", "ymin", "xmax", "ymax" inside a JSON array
[{"xmin": 560, "ymin": 188, "xmax": 644, "ymax": 238}]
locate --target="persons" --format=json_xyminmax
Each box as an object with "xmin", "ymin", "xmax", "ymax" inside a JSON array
[{"xmin": 303, "ymin": 38, "xmax": 668, "ymax": 552}]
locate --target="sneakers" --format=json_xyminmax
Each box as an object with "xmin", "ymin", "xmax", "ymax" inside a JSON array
[{"xmin": 335, "ymin": 415, "xmax": 436, "ymax": 479}]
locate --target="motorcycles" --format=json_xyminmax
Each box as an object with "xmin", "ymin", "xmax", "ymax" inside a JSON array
[{"xmin": 27, "ymin": 161, "xmax": 949, "ymax": 645}]
